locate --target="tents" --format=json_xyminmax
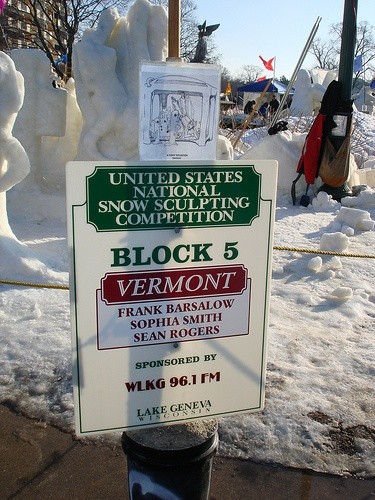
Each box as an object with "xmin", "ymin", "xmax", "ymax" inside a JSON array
[{"xmin": 236, "ymin": 78, "xmax": 295, "ymax": 108}]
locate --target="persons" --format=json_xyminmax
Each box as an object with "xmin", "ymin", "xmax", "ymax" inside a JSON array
[
  {"xmin": 258, "ymin": 102, "xmax": 268, "ymax": 118},
  {"xmin": 244, "ymin": 100, "xmax": 258, "ymax": 117},
  {"xmin": 269, "ymin": 95, "xmax": 280, "ymax": 113}
]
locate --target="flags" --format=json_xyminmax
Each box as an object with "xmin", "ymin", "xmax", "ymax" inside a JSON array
[{"xmin": 352, "ymin": 54, "xmax": 363, "ymax": 72}]
